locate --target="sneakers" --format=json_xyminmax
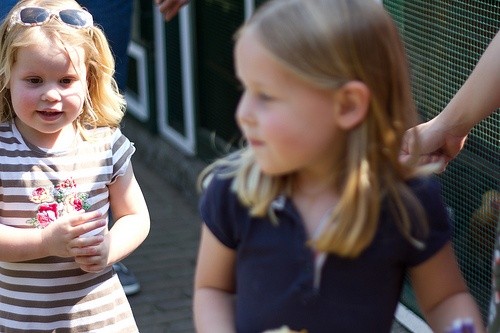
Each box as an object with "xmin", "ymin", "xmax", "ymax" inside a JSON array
[{"xmin": 111, "ymin": 261, "xmax": 140, "ymax": 294}]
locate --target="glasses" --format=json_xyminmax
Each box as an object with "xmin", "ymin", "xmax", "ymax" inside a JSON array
[{"xmin": 3, "ymin": 5, "xmax": 94, "ymax": 40}]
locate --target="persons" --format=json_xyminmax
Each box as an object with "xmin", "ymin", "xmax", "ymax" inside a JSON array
[
  {"xmin": 0, "ymin": 0, "xmax": 151, "ymax": 333},
  {"xmin": 0, "ymin": 0, "xmax": 190, "ymax": 296},
  {"xmin": 194, "ymin": 0, "xmax": 500, "ymax": 333},
  {"xmin": 398, "ymin": 30, "xmax": 500, "ymax": 176}
]
